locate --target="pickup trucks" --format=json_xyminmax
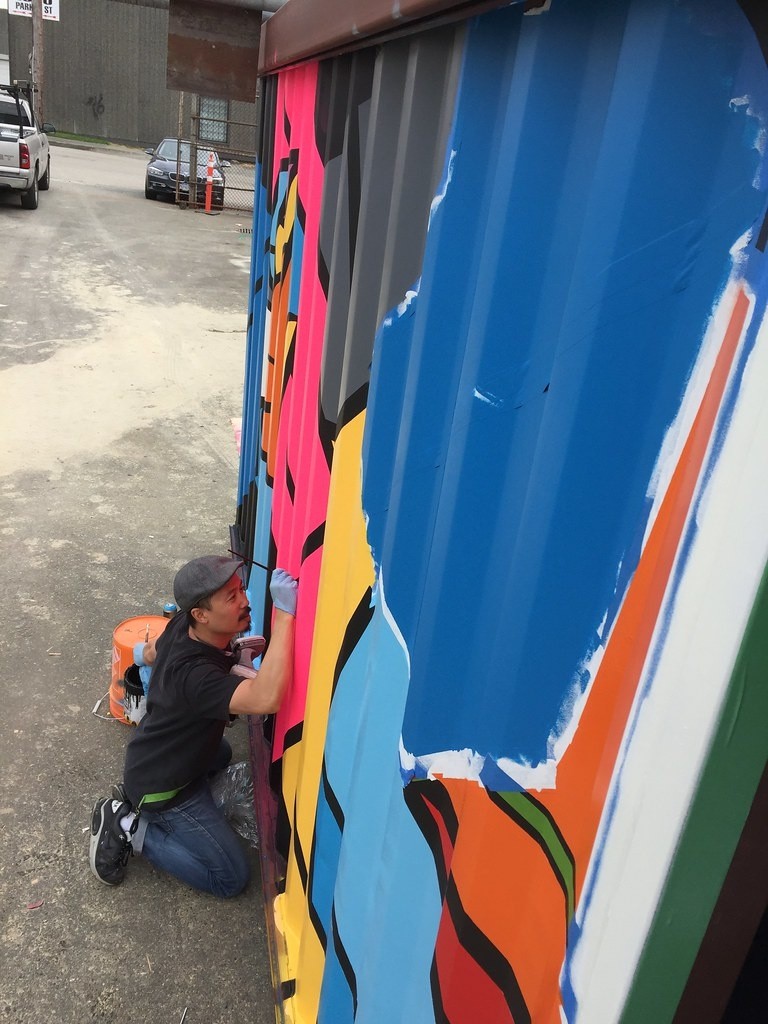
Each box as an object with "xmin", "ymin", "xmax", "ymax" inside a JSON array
[{"xmin": 0, "ymin": 94, "xmax": 56, "ymax": 209}]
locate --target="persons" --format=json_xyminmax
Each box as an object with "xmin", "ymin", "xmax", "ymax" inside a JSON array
[{"xmin": 84, "ymin": 554, "xmax": 299, "ymax": 894}]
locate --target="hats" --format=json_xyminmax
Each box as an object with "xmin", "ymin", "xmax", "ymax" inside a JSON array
[{"xmin": 173, "ymin": 555, "xmax": 245, "ymax": 615}]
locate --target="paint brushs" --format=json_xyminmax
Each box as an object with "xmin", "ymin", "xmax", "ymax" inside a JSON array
[
  {"xmin": 227, "ymin": 549, "xmax": 273, "ymax": 573},
  {"xmin": 145, "ymin": 624, "xmax": 150, "ymax": 643}
]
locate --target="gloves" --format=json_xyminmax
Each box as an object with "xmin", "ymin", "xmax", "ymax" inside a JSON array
[
  {"xmin": 269, "ymin": 568, "xmax": 298, "ymax": 619},
  {"xmin": 133, "ymin": 642, "xmax": 147, "ymax": 667}
]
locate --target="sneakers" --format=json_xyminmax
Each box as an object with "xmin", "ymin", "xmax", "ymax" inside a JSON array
[
  {"xmin": 89, "ymin": 795, "xmax": 132, "ymax": 887},
  {"xmin": 111, "ymin": 781, "xmax": 131, "ymax": 805}
]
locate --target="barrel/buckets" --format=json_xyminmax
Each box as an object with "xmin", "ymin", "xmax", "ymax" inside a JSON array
[{"xmin": 93, "ymin": 614, "xmax": 176, "ymax": 726}]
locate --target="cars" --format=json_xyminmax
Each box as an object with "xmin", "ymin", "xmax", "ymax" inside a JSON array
[{"xmin": 144, "ymin": 137, "xmax": 231, "ymax": 211}]
